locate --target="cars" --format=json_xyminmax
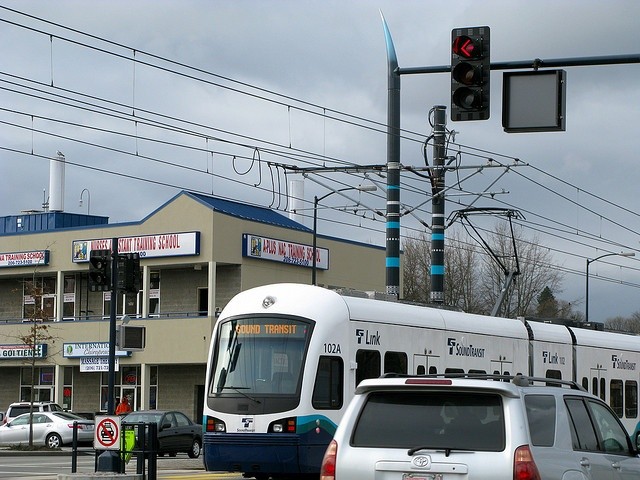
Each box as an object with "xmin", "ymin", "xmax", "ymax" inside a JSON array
[
  {"xmin": 119, "ymin": 410, "xmax": 202, "ymax": 458},
  {"xmin": 0, "ymin": 412, "xmax": 94, "ymax": 450},
  {"xmin": 0, "ymin": 410, "xmax": 5, "ymax": 425}
]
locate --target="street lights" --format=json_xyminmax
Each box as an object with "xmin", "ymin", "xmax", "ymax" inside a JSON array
[{"xmin": 582, "ymin": 250, "xmax": 638, "ymax": 320}]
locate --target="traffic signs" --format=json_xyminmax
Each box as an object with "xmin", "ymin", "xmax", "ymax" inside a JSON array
[{"xmin": 311, "ymin": 184, "xmax": 380, "ymax": 288}]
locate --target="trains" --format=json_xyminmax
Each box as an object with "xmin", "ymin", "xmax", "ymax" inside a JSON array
[{"xmin": 202, "ymin": 283, "xmax": 639, "ymax": 479}]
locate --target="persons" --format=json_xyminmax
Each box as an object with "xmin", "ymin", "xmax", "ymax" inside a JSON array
[{"xmin": 115, "ymin": 397, "xmax": 132, "ymax": 415}]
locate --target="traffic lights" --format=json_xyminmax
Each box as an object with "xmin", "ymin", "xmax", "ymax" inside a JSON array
[
  {"xmin": 450, "ymin": 25, "xmax": 493, "ymax": 122},
  {"xmin": 90, "ymin": 249, "xmax": 113, "ymax": 294}
]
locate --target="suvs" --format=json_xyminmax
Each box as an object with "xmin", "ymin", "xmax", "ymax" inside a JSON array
[
  {"xmin": 5, "ymin": 402, "xmax": 68, "ymax": 423},
  {"xmin": 318, "ymin": 371, "xmax": 639, "ymax": 480}
]
